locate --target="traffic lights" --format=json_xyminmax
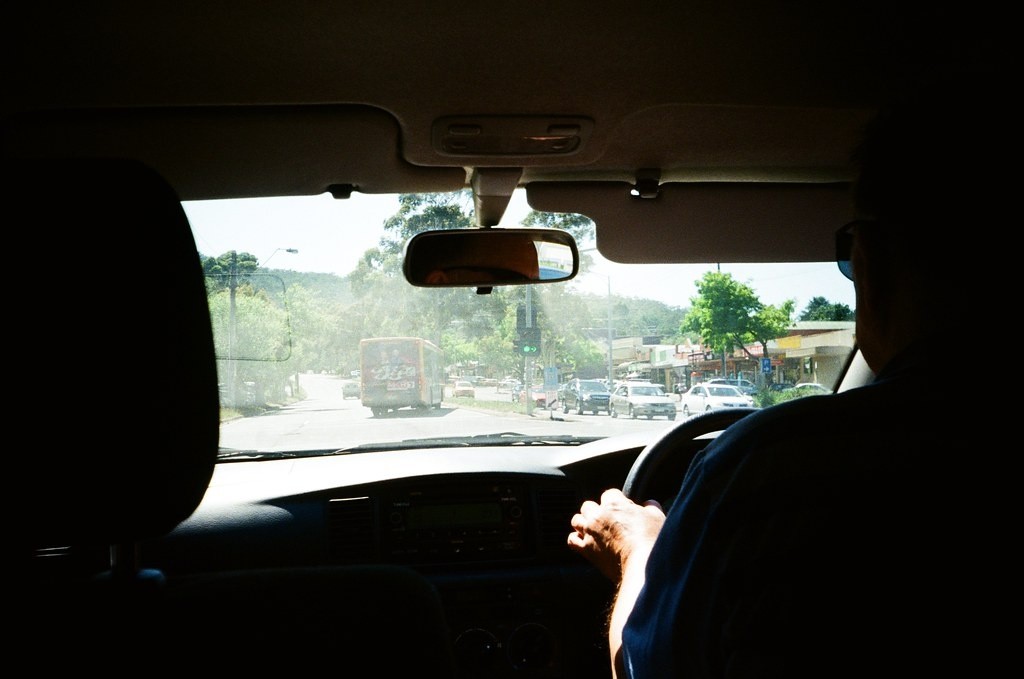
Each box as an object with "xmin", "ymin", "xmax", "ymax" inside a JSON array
[{"xmin": 519, "ymin": 327, "xmax": 541, "ymax": 356}]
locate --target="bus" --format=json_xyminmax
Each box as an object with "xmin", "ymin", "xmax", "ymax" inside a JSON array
[{"xmin": 359, "ymin": 337, "xmax": 445, "ymax": 416}]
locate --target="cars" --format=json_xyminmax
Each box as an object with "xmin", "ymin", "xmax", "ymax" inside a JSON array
[
  {"xmin": 679, "ymin": 383, "xmax": 750, "ymax": 418},
  {"xmin": 589, "ymin": 374, "xmax": 832, "ymax": 405},
  {"xmin": 609, "ymin": 381, "xmax": 676, "ymax": 420},
  {"xmin": 305, "ymin": 367, "xmax": 360, "ymax": 378},
  {"xmin": 341, "ymin": 381, "xmax": 361, "ymax": 401},
  {"xmin": 561, "ymin": 377, "xmax": 614, "ymax": 415},
  {"xmin": 444, "ymin": 371, "xmax": 563, "ymax": 410}
]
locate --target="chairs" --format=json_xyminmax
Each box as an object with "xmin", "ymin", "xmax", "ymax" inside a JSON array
[{"xmin": 0, "ymin": 155, "xmax": 223, "ymax": 678}]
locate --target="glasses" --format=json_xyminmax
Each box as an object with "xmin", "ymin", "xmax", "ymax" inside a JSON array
[
  {"xmin": 447, "ymin": 265, "xmax": 530, "ymax": 282},
  {"xmin": 835, "ymin": 218, "xmax": 855, "ymax": 281}
]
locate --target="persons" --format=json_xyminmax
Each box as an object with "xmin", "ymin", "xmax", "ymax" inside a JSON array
[{"xmin": 568, "ymin": 94, "xmax": 1020, "ymax": 679}]
